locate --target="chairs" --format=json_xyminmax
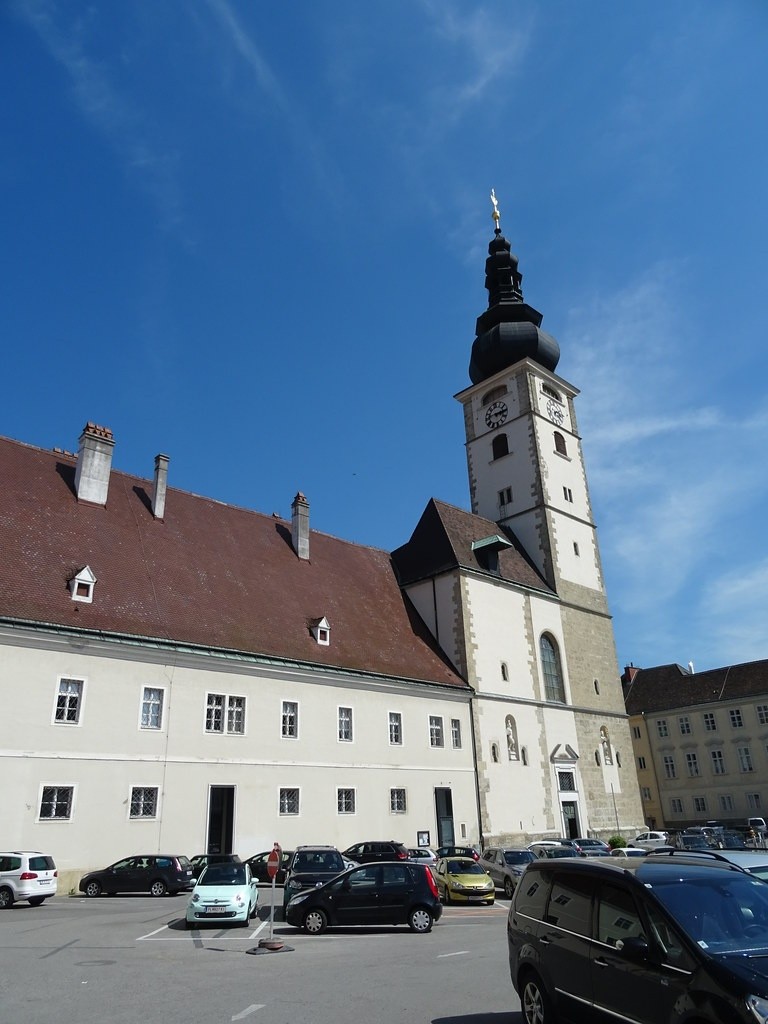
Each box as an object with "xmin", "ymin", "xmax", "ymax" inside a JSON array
[
  {"xmin": 138, "ymin": 860, "xmax": 144, "ymax": 868},
  {"xmin": 144, "ymin": 860, "xmax": 150, "ymax": 868},
  {"xmin": 323, "ymin": 854, "xmax": 334, "ymax": 869},
  {"xmin": 294, "ymin": 855, "xmax": 309, "ymax": 872}
]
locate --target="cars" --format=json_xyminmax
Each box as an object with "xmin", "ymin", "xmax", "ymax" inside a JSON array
[
  {"xmin": 524, "ymin": 841, "xmax": 561, "ymax": 851},
  {"xmin": 436, "ymin": 847, "xmax": 479, "ymax": 861},
  {"xmin": 432, "ymin": 856, "xmax": 495, "ymax": 905},
  {"xmin": 610, "ymin": 848, "xmax": 649, "ymax": 857},
  {"xmin": 579, "ymin": 849, "xmax": 608, "ymax": 856},
  {"xmin": 190, "ymin": 854, "xmax": 241, "ymax": 881},
  {"xmin": 543, "ymin": 838, "xmax": 574, "ymax": 847},
  {"xmin": 626, "ymin": 832, "xmax": 669, "ymax": 845},
  {"xmin": 533, "ymin": 845, "xmax": 579, "ymax": 859},
  {"xmin": 661, "ymin": 826, "xmax": 748, "ymax": 847},
  {"xmin": 408, "ymin": 848, "xmax": 440, "ymax": 867},
  {"xmin": 186, "ymin": 862, "xmax": 258, "ymax": 927},
  {"xmin": 286, "ymin": 862, "xmax": 443, "ymax": 935},
  {"xmin": 572, "ymin": 839, "xmax": 611, "ymax": 852},
  {"xmin": 79, "ymin": 854, "xmax": 194, "ymax": 897},
  {"xmin": 243, "ymin": 851, "xmax": 295, "ymax": 884}
]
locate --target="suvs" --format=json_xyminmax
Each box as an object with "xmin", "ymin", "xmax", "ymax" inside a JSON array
[
  {"xmin": 508, "ymin": 847, "xmax": 768, "ymax": 1024},
  {"xmin": 0, "ymin": 850, "xmax": 58, "ymax": 908},
  {"xmin": 283, "ymin": 845, "xmax": 353, "ymax": 915},
  {"xmin": 343, "ymin": 840, "xmax": 411, "ymax": 871},
  {"xmin": 478, "ymin": 846, "xmax": 539, "ymax": 900}
]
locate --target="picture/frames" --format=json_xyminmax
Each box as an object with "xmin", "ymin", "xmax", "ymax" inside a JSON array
[{"xmin": 417, "ymin": 831, "xmax": 430, "ymax": 846}]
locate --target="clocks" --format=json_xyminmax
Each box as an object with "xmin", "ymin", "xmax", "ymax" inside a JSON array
[
  {"xmin": 546, "ymin": 399, "xmax": 564, "ymax": 426},
  {"xmin": 485, "ymin": 402, "xmax": 508, "ymax": 429}
]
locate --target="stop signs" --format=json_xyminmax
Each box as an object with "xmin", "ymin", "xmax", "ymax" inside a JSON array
[{"xmin": 267, "ymin": 851, "xmax": 280, "ymax": 879}]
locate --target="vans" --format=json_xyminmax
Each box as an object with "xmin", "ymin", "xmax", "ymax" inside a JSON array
[{"xmin": 747, "ymin": 818, "xmax": 767, "ymax": 839}]
